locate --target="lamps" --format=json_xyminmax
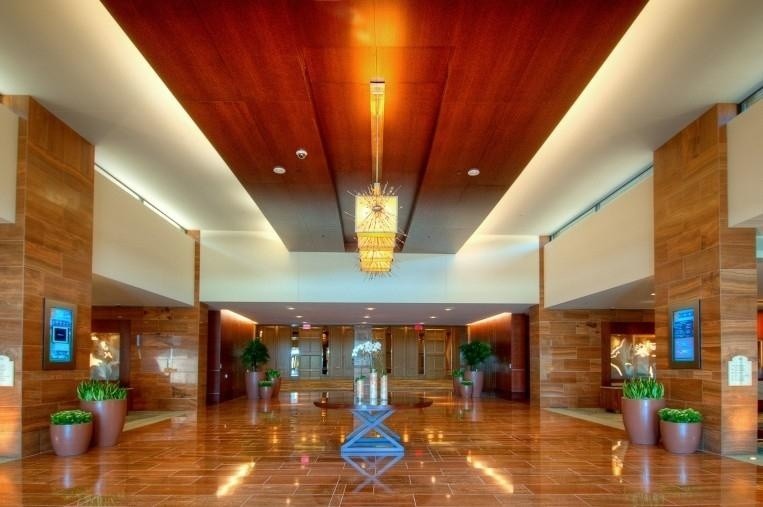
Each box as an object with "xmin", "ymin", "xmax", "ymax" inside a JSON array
[{"xmin": 354, "ymin": 80, "xmax": 399, "ymax": 272}]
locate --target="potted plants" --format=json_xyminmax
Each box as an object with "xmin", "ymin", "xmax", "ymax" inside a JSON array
[
  {"xmin": 620, "ymin": 375, "xmax": 705, "ymax": 456},
  {"xmin": 238, "ymin": 338, "xmax": 281, "ymax": 400},
  {"xmin": 452, "ymin": 340, "xmax": 492, "ymax": 398},
  {"xmin": 50, "ymin": 378, "xmax": 129, "ymax": 457}
]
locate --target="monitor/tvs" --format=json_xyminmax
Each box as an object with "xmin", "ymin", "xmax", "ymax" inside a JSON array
[
  {"xmin": 43, "ymin": 297, "xmax": 77, "ymax": 370},
  {"xmin": 667, "ymin": 298, "xmax": 701, "ymax": 369}
]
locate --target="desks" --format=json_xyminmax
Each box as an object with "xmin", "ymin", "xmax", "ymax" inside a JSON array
[{"xmin": 339, "ymin": 402, "xmax": 405, "ymax": 455}]
